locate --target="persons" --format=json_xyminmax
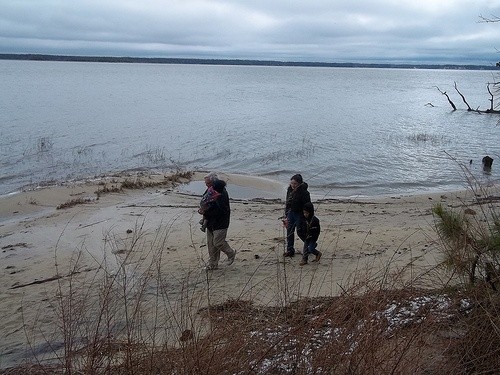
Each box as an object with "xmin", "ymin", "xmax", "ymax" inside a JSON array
[
  {"xmin": 298, "ymin": 203, "xmax": 322, "ymax": 266},
  {"xmin": 282, "ymin": 173, "xmax": 319, "ymax": 257},
  {"xmin": 198, "ymin": 172, "xmax": 237, "ymax": 270}
]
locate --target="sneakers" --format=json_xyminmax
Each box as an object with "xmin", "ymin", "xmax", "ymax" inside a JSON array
[
  {"xmin": 204, "ymin": 265, "xmax": 219, "ymax": 269},
  {"xmin": 226, "ymin": 249, "xmax": 236, "ymax": 265}
]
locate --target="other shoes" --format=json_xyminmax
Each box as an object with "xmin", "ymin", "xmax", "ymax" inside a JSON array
[
  {"xmin": 283, "ymin": 253, "xmax": 288, "ymax": 257},
  {"xmin": 200, "ymin": 227, "xmax": 205, "ymax": 232},
  {"xmin": 315, "ymin": 243, "xmax": 317, "ymax": 248},
  {"xmin": 300, "ymin": 261, "xmax": 307, "ymax": 265},
  {"xmin": 199, "ymin": 219, "xmax": 204, "ymax": 225},
  {"xmin": 315, "ymin": 252, "xmax": 322, "ymax": 261}
]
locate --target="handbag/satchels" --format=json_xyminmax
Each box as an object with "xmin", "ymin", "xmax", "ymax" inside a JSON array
[{"xmin": 282, "ymin": 217, "xmax": 297, "ymax": 229}]
